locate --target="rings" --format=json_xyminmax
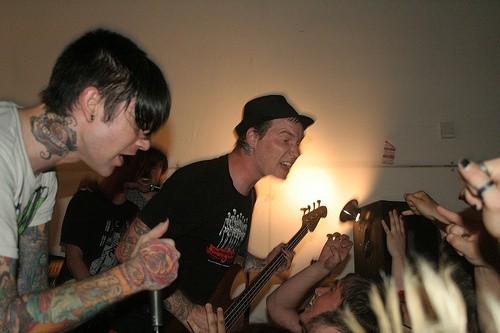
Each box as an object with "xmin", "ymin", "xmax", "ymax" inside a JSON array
[{"xmin": 479, "ymin": 180, "xmax": 494, "ymax": 192}]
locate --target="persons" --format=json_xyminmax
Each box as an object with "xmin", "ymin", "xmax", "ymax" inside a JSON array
[
  {"xmin": 0, "ymin": 28, "xmax": 181, "ymax": 333},
  {"xmin": 55, "ymin": 149, "xmax": 167, "ymax": 333},
  {"xmin": 266, "ymin": 156, "xmax": 500, "ymax": 332},
  {"xmin": 116, "ymin": 94, "xmax": 315, "ymax": 333}
]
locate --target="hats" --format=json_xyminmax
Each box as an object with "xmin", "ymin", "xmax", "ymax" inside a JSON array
[{"xmin": 235, "ymin": 95, "xmax": 315, "ymax": 135}]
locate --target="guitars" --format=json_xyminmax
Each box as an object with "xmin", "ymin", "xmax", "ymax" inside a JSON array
[{"xmin": 223, "ymin": 200, "xmax": 328, "ymax": 333}]
